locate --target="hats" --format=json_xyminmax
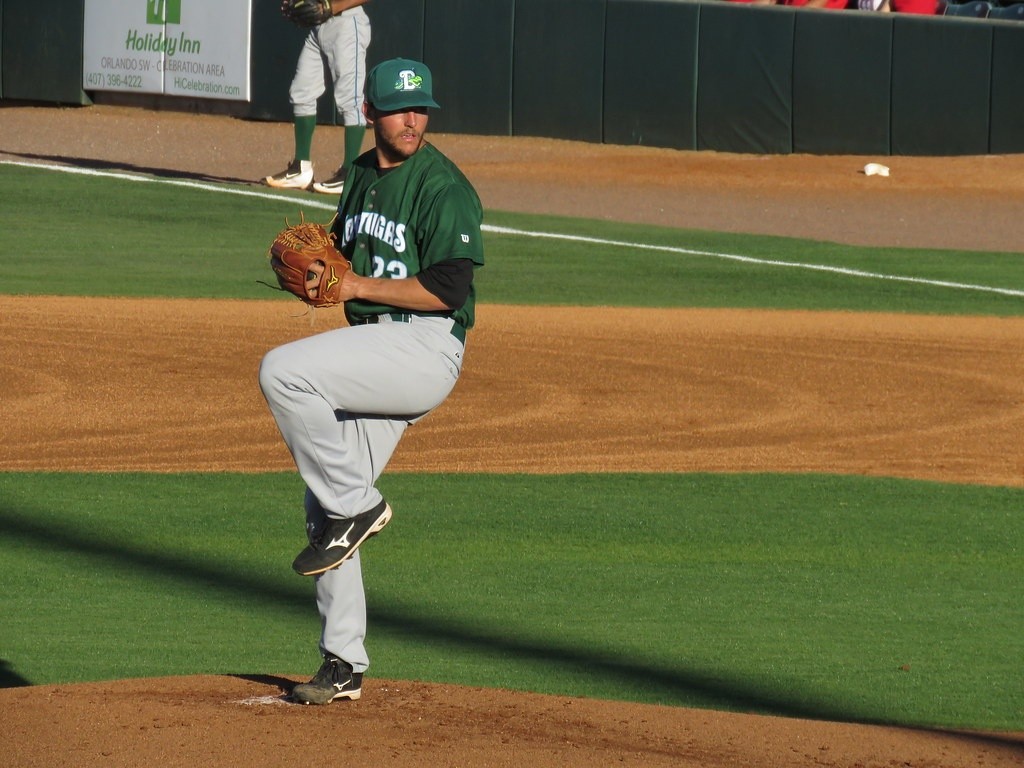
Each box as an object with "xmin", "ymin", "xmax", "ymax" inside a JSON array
[{"xmin": 364, "ymin": 57, "xmax": 441, "ymax": 112}]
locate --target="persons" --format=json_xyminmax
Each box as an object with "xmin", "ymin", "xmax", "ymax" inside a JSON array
[
  {"xmin": 726, "ymin": 0, "xmax": 946, "ymax": 16},
  {"xmin": 265, "ymin": 0, "xmax": 371, "ymax": 193},
  {"xmin": 258, "ymin": 56, "xmax": 486, "ymax": 709}
]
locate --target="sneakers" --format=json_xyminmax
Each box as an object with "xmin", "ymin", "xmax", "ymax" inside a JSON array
[
  {"xmin": 261, "ymin": 160, "xmax": 314, "ymax": 190},
  {"xmin": 293, "ymin": 653, "xmax": 363, "ymax": 706},
  {"xmin": 292, "ymin": 498, "xmax": 393, "ymax": 577},
  {"xmin": 312, "ymin": 167, "xmax": 347, "ymax": 193}
]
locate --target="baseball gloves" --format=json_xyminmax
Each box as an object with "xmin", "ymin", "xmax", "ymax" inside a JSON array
[
  {"xmin": 282, "ymin": 0, "xmax": 334, "ymax": 26},
  {"xmin": 268, "ymin": 221, "xmax": 350, "ymax": 308}
]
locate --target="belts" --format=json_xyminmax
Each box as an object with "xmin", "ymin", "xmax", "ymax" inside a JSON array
[{"xmin": 363, "ymin": 312, "xmax": 466, "ymax": 347}]
